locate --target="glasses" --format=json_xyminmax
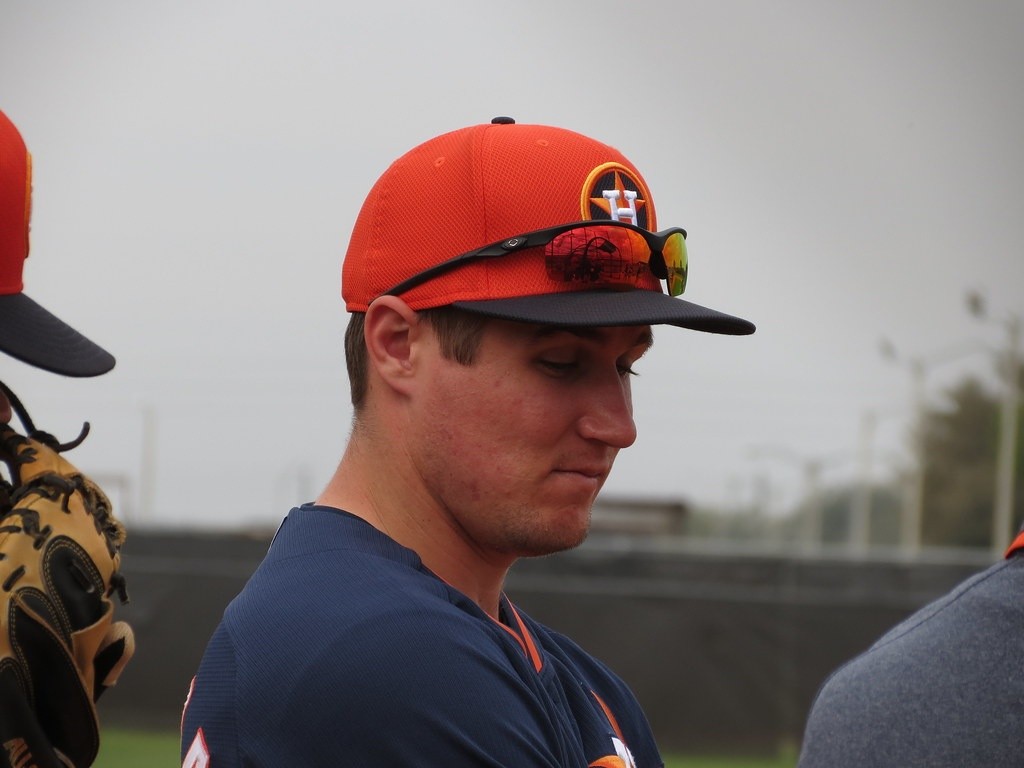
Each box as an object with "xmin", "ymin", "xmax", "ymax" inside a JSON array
[{"xmin": 368, "ymin": 220, "xmax": 690, "ymax": 296}]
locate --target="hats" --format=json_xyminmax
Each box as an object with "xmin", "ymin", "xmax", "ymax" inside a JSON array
[
  {"xmin": 344, "ymin": 117, "xmax": 757, "ymax": 338},
  {"xmin": 1, "ymin": 110, "xmax": 117, "ymax": 378}
]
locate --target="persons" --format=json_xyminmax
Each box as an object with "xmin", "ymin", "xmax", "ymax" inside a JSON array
[
  {"xmin": 174, "ymin": 112, "xmax": 762, "ymax": 765},
  {"xmin": 796, "ymin": 498, "xmax": 1023, "ymax": 768},
  {"xmin": 0, "ymin": 107, "xmax": 140, "ymax": 767}
]
